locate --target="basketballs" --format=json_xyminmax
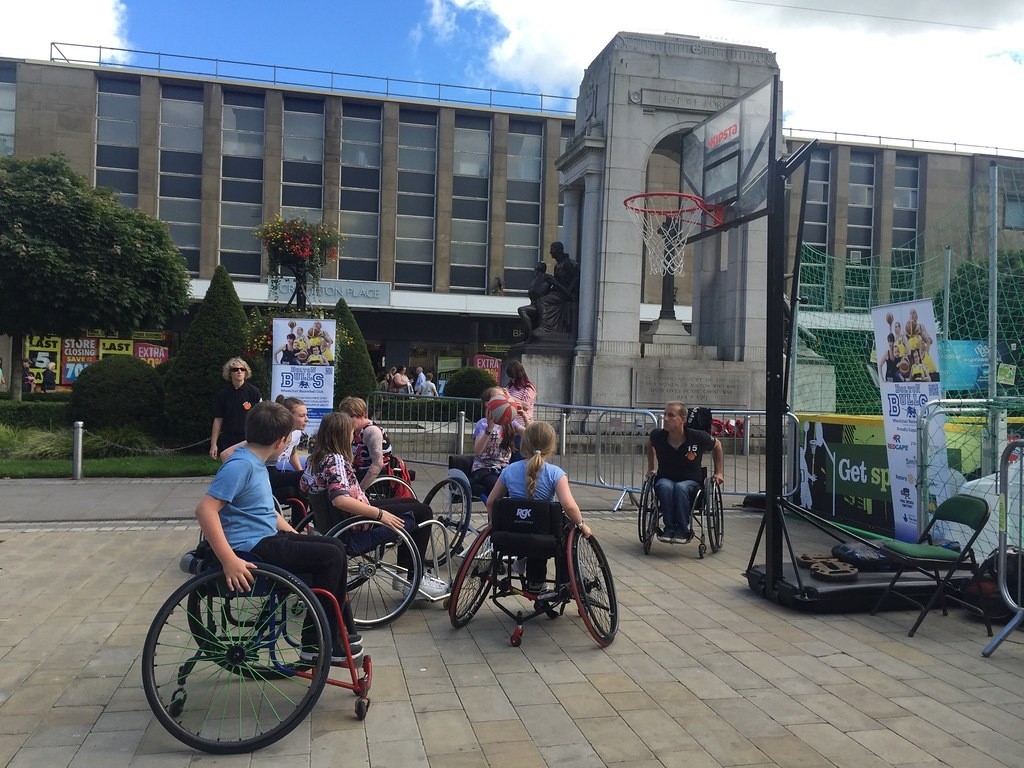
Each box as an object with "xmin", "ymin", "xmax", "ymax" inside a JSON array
[
  {"xmin": 308, "ymin": 327, "xmax": 319, "ymax": 337},
  {"xmin": 886, "ymin": 312, "xmax": 894, "ymax": 324},
  {"xmin": 298, "ymin": 353, "xmax": 307, "ymax": 361},
  {"xmin": 486, "ymin": 394, "xmax": 518, "ymax": 426},
  {"xmin": 905, "ymin": 320, "xmax": 917, "ymax": 335},
  {"xmin": 288, "ymin": 320, "xmax": 296, "ymax": 328},
  {"xmin": 899, "ymin": 363, "xmax": 910, "ymax": 373}
]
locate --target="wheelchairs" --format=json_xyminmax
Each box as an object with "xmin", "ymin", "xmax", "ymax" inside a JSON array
[
  {"xmin": 448, "ymin": 498, "xmax": 620, "ymax": 648},
  {"xmin": 422, "ymin": 455, "xmax": 517, "ymax": 566},
  {"xmin": 272, "ymin": 488, "xmax": 315, "ymax": 536},
  {"xmin": 638, "ymin": 467, "xmax": 724, "ymax": 559},
  {"xmin": 141, "ymin": 529, "xmax": 373, "ymax": 754},
  {"xmin": 293, "ymin": 490, "xmax": 454, "ymax": 627},
  {"xmin": 355, "ymin": 457, "xmax": 418, "ymax": 548}
]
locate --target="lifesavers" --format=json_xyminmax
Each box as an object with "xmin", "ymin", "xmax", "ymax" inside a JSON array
[
  {"xmin": 726, "ymin": 418, "xmax": 744, "ymax": 437},
  {"xmin": 711, "ymin": 419, "xmax": 725, "ymax": 435}
]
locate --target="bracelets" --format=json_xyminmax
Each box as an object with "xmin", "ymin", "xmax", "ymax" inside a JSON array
[
  {"xmin": 376, "ymin": 508, "xmax": 382, "ymax": 521},
  {"xmin": 578, "ymin": 519, "xmax": 585, "ymax": 530},
  {"xmin": 485, "ymin": 429, "xmax": 491, "ymax": 436},
  {"xmin": 3, "ymin": 383, "xmax": 6, "ymax": 384}
]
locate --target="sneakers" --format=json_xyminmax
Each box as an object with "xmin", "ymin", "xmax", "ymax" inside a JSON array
[
  {"xmin": 528, "ymin": 580, "xmax": 548, "ymax": 593},
  {"xmin": 672, "ymin": 526, "xmax": 688, "ymax": 543},
  {"xmin": 339, "ymin": 632, "xmax": 362, "ymax": 646},
  {"xmin": 401, "ymin": 577, "xmax": 447, "ymax": 600},
  {"xmin": 659, "ymin": 528, "xmax": 674, "ymax": 541},
  {"xmin": 392, "ymin": 572, "xmax": 408, "ymax": 590},
  {"xmin": 300, "ymin": 641, "xmax": 364, "ymax": 662}
]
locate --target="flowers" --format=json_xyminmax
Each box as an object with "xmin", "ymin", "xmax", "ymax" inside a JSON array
[{"xmin": 240, "ymin": 207, "xmax": 354, "ymax": 359}]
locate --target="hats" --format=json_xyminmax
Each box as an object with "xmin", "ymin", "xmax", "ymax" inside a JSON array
[{"xmin": 22, "ymin": 357, "xmax": 32, "ymax": 364}]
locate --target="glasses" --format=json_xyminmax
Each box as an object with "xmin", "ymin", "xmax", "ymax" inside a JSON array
[{"xmin": 229, "ymin": 367, "xmax": 246, "ymax": 373}]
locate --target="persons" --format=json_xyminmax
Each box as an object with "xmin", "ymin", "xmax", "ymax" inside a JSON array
[
  {"xmin": 274, "ymin": 322, "xmax": 333, "ymax": 366},
  {"xmin": 209, "ymin": 356, "xmax": 263, "ymax": 465},
  {"xmin": 300, "ymin": 411, "xmax": 448, "ymax": 601},
  {"xmin": 220, "ymin": 394, "xmax": 308, "ymax": 535},
  {"xmin": 22, "ymin": 358, "xmax": 36, "ymax": 393},
  {"xmin": 526, "ymin": 242, "xmax": 580, "ymax": 336},
  {"xmin": 645, "ymin": 400, "xmax": 724, "ymax": 543},
  {"xmin": 194, "ymin": 400, "xmax": 365, "ymax": 662},
  {"xmin": 379, "ymin": 364, "xmax": 439, "ymax": 401},
  {"xmin": 879, "ymin": 309, "xmax": 940, "ymax": 382},
  {"xmin": 0, "ymin": 360, "xmax": 8, "ymax": 390},
  {"xmin": 504, "ymin": 360, "xmax": 537, "ymax": 465},
  {"xmin": 41, "ymin": 362, "xmax": 56, "ymax": 394},
  {"xmin": 471, "ymin": 385, "xmax": 531, "ymax": 509},
  {"xmin": 518, "ymin": 261, "xmax": 576, "ymax": 344},
  {"xmin": 338, "ymin": 396, "xmax": 393, "ymax": 500},
  {"xmin": 486, "ymin": 421, "xmax": 593, "ymax": 594}
]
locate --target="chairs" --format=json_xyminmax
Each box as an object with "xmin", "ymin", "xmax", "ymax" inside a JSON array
[{"xmin": 870, "ymin": 494, "xmax": 994, "ymax": 638}]
